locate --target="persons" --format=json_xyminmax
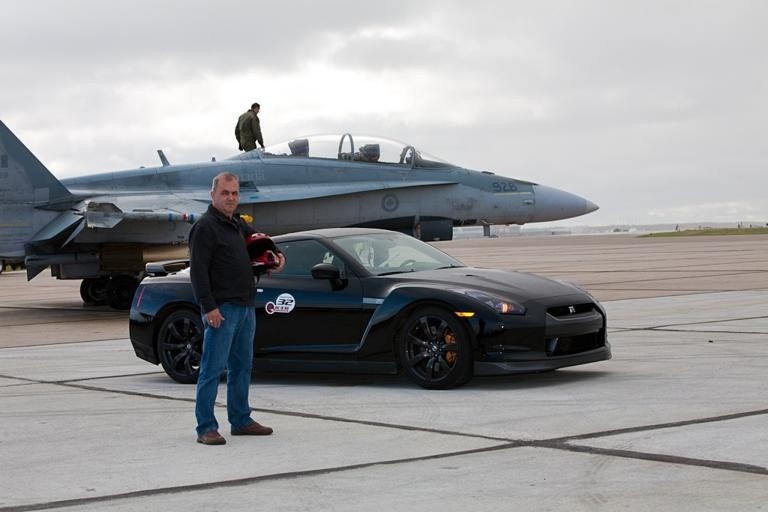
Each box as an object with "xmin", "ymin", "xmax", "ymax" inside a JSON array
[
  {"xmin": 188, "ymin": 174, "xmax": 285, "ymax": 446},
  {"xmin": 234, "ymin": 103, "xmax": 265, "ymax": 154}
]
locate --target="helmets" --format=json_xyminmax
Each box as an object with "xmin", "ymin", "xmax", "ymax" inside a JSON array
[{"xmin": 247, "ymin": 232, "xmax": 279, "ymax": 267}]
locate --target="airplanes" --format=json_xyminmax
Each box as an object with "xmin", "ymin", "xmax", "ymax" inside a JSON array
[{"xmin": 2, "ymin": 120, "xmax": 599, "ymax": 303}]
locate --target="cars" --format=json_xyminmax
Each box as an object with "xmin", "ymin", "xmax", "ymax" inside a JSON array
[{"xmin": 129, "ymin": 224, "xmax": 613, "ymax": 389}]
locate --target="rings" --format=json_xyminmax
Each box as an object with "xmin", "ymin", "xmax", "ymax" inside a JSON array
[{"xmin": 209, "ymin": 320, "xmax": 213, "ymax": 324}]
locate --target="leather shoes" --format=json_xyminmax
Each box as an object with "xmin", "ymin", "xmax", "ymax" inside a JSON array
[
  {"xmin": 230, "ymin": 422, "xmax": 273, "ymax": 435},
  {"xmin": 198, "ymin": 430, "xmax": 225, "ymax": 444}
]
